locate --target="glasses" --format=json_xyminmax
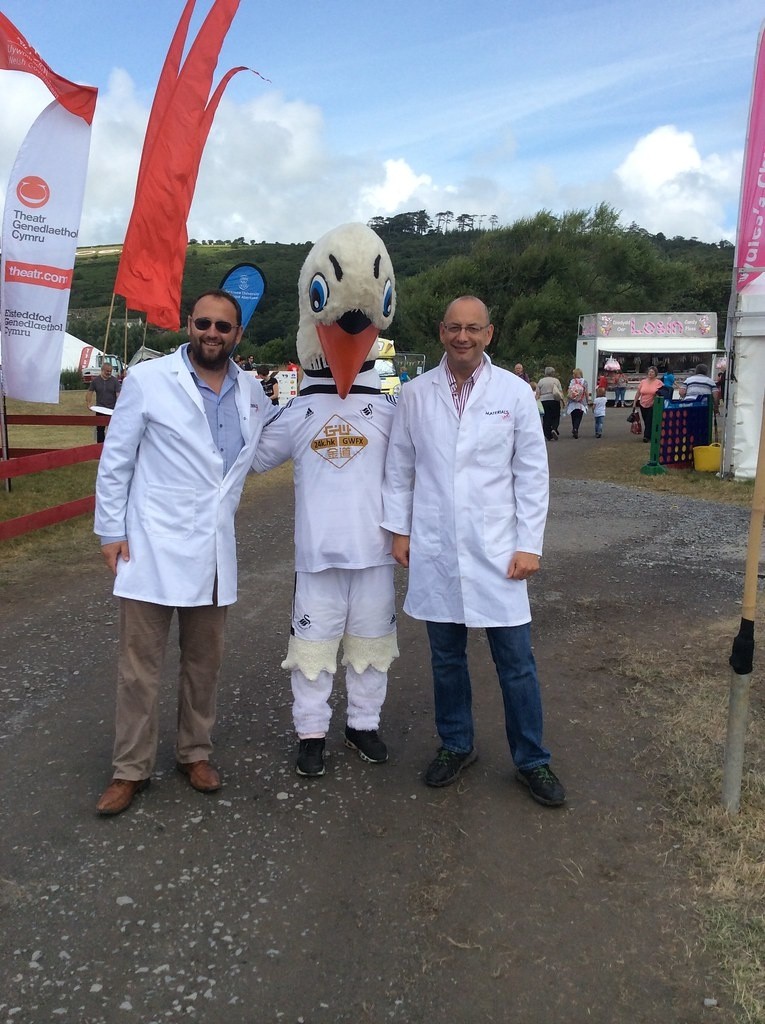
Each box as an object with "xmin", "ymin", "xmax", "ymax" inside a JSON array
[
  {"xmin": 441, "ymin": 321, "xmax": 494, "ymax": 335},
  {"xmin": 187, "ymin": 317, "xmax": 239, "ymax": 335}
]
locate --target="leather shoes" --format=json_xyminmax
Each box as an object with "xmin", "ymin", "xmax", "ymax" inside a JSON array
[
  {"xmin": 172, "ymin": 756, "xmax": 224, "ymax": 793},
  {"xmin": 95, "ymin": 773, "xmax": 149, "ymax": 817}
]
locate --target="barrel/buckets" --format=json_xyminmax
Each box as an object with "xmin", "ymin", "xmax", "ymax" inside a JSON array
[{"xmin": 693, "ymin": 442, "xmax": 723, "ymax": 471}]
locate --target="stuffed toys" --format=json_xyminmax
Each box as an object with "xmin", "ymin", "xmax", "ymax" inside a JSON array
[{"xmin": 247, "ymin": 222, "xmax": 400, "ymax": 775}]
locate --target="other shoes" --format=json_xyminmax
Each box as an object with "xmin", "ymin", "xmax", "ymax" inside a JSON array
[
  {"xmin": 551, "ymin": 430, "xmax": 558, "ymax": 440},
  {"xmin": 614, "ymin": 404, "xmax": 617, "ymax": 408},
  {"xmin": 573, "ymin": 428, "xmax": 578, "ymax": 439},
  {"xmin": 595, "ymin": 433, "xmax": 601, "ymax": 438},
  {"xmin": 621, "ymin": 404, "xmax": 625, "ymax": 408}
]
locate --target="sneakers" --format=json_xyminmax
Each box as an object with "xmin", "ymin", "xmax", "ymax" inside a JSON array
[
  {"xmin": 343, "ymin": 722, "xmax": 390, "ymax": 763},
  {"xmin": 424, "ymin": 745, "xmax": 478, "ymax": 788},
  {"xmin": 294, "ymin": 736, "xmax": 326, "ymax": 776},
  {"xmin": 515, "ymin": 761, "xmax": 567, "ymax": 806}
]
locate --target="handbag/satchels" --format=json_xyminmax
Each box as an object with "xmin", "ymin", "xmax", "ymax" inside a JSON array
[
  {"xmin": 627, "ymin": 407, "xmax": 640, "ymax": 424},
  {"xmin": 630, "ymin": 421, "xmax": 642, "ymax": 435},
  {"xmin": 536, "ymin": 399, "xmax": 545, "ymax": 416},
  {"xmin": 693, "ymin": 442, "xmax": 722, "ymax": 472},
  {"xmin": 552, "ymin": 382, "xmax": 562, "ymax": 402}
]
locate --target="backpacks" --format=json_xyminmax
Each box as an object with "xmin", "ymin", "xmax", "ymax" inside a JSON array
[
  {"xmin": 567, "ymin": 378, "xmax": 586, "ymax": 403},
  {"xmin": 616, "ymin": 376, "xmax": 628, "ymax": 389}
]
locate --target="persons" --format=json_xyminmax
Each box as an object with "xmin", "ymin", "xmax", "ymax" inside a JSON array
[
  {"xmin": 566, "ymin": 368, "xmax": 589, "ymax": 439},
  {"xmin": 400, "ymin": 367, "xmax": 411, "ymax": 384},
  {"xmin": 633, "ymin": 366, "xmax": 665, "ymax": 443},
  {"xmin": 662, "ymin": 366, "xmax": 680, "ymax": 388},
  {"xmin": 592, "ymin": 387, "xmax": 607, "ymax": 438},
  {"xmin": 233, "ymin": 353, "xmax": 254, "ymax": 371},
  {"xmin": 679, "ymin": 364, "xmax": 720, "ymax": 414},
  {"xmin": 535, "ymin": 366, "xmax": 565, "ymax": 441},
  {"xmin": 596, "ymin": 371, "xmax": 607, "ymax": 389},
  {"xmin": 286, "ymin": 360, "xmax": 299, "ymax": 371},
  {"xmin": 514, "ymin": 363, "xmax": 537, "ymax": 391},
  {"xmin": 256, "ymin": 366, "xmax": 279, "ymax": 406},
  {"xmin": 93, "ymin": 289, "xmax": 283, "ymax": 815},
  {"xmin": 379, "ymin": 295, "xmax": 567, "ymax": 805},
  {"xmin": 613, "ymin": 370, "xmax": 629, "ymax": 408},
  {"xmin": 87, "ymin": 362, "xmax": 122, "ymax": 444}
]
